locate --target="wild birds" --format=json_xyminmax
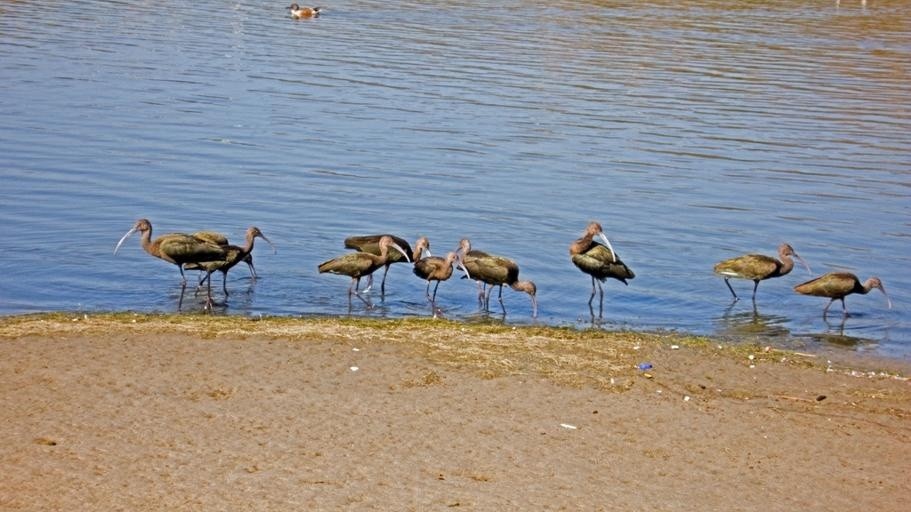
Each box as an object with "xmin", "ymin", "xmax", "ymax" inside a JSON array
[
  {"xmin": 793, "ymin": 271, "xmax": 893, "ymax": 314},
  {"xmin": 714, "ymin": 243, "xmax": 801, "ymax": 298},
  {"xmin": 112, "ymin": 216, "xmax": 278, "ymax": 313},
  {"xmin": 568, "ymin": 221, "xmax": 636, "ymax": 305},
  {"xmin": 315, "ymin": 233, "xmax": 539, "ymax": 318}
]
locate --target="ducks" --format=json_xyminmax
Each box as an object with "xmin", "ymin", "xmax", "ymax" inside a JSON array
[{"xmin": 285, "ymin": 3, "xmax": 322, "ymax": 19}]
